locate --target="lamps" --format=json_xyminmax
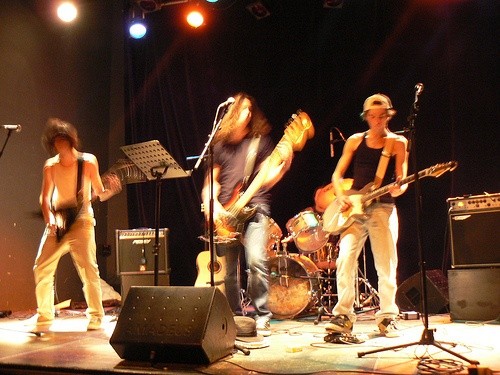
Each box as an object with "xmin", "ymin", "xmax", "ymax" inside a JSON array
[{"xmin": 127, "ymin": 0, "xmax": 165, "ymax": 38}]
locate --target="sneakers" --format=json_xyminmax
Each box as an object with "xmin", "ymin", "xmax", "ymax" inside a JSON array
[
  {"xmin": 87, "ymin": 315, "xmax": 102, "ymax": 330},
  {"xmin": 324, "ymin": 314, "xmax": 353, "ymax": 333},
  {"xmin": 378, "ymin": 318, "xmax": 402, "ymax": 337},
  {"xmin": 255, "ymin": 316, "xmax": 271, "ymax": 336},
  {"xmin": 28, "ymin": 321, "xmax": 51, "ymax": 333}
]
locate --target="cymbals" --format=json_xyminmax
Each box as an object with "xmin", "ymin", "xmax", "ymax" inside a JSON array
[{"xmin": 315, "ymin": 176, "xmax": 354, "ymax": 213}]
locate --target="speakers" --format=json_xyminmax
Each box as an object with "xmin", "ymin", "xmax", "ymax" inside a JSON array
[
  {"xmin": 394, "ymin": 268, "xmax": 450, "ymax": 315},
  {"xmin": 116, "ymin": 229, "xmax": 168, "ymax": 275},
  {"xmin": 446, "ymin": 193, "xmax": 500, "ymax": 268},
  {"xmin": 110, "ymin": 285, "xmax": 237, "ymax": 366}
]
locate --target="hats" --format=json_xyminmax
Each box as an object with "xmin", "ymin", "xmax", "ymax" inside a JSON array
[{"xmin": 363, "ymin": 94, "xmax": 391, "ymax": 112}]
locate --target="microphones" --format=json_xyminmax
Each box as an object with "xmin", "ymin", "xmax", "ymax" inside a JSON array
[
  {"xmin": 413, "ymin": 83, "xmax": 424, "ymax": 105},
  {"xmin": 219, "ymin": 96, "xmax": 236, "ymax": 108},
  {"xmin": 329, "ymin": 130, "xmax": 334, "ymax": 158},
  {"xmin": 1, "ymin": 124, "xmax": 22, "ymax": 133}
]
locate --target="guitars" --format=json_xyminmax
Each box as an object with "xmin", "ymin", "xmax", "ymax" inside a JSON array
[
  {"xmin": 194, "ymin": 203, "xmax": 227, "ymax": 296},
  {"xmin": 213, "ymin": 109, "xmax": 315, "ymax": 247},
  {"xmin": 322, "ymin": 157, "xmax": 458, "ymax": 235},
  {"xmin": 26, "ymin": 156, "xmax": 143, "ymax": 246}
]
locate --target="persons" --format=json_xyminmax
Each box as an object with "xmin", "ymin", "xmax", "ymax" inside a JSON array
[
  {"xmin": 201, "ymin": 94, "xmax": 293, "ymax": 338},
  {"xmin": 325, "ymin": 94, "xmax": 408, "ymax": 338},
  {"xmin": 22, "ymin": 119, "xmax": 122, "ymax": 333}
]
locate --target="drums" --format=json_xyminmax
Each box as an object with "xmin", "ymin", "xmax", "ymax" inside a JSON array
[
  {"xmin": 305, "ymin": 242, "xmax": 339, "ymax": 272},
  {"xmin": 286, "ymin": 208, "xmax": 329, "ymax": 254},
  {"xmin": 267, "ymin": 254, "xmax": 320, "ymax": 320},
  {"xmin": 239, "ymin": 215, "xmax": 283, "ymax": 253}
]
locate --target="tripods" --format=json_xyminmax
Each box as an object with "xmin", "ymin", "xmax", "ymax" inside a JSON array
[
  {"xmin": 307, "ymin": 235, "xmax": 380, "ymax": 325},
  {"xmin": 356, "ymin": 102, "xmax": 480, "ymax": 365}
]
